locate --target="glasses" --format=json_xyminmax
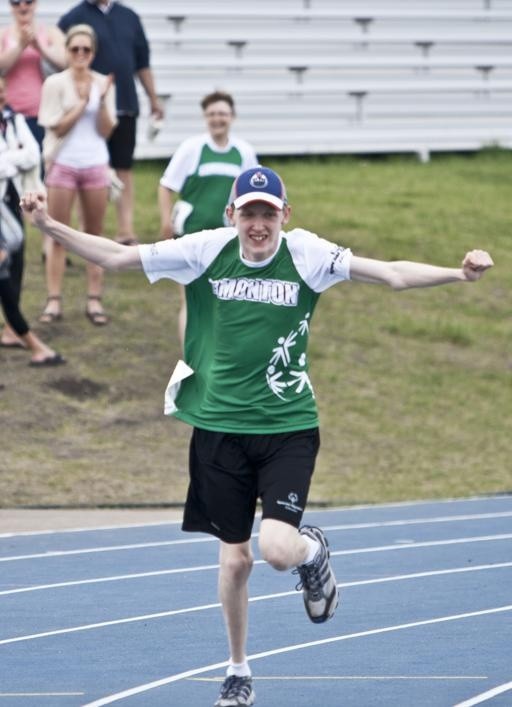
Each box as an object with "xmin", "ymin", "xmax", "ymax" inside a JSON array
[
  {"xmin": 9, "ymin": 1, "xmax": 36, "ymax": 5},
  {"xmin": 69, "ymin": 45, "xmax": 93, "ymax": 55}
]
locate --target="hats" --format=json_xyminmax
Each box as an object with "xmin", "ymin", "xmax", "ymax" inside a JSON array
[{"xmin": 233, "ymin": 166, "xmax": 286, "ymax": 209}]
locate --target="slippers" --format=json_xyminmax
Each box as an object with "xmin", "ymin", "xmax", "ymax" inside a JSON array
[
  {"xmin": 3, "ymin": 338, "xmax": 30, "ymax": 350},
  {"xmin": 29, "ymin": 351, "xmax": 68, "ymax": 367}
]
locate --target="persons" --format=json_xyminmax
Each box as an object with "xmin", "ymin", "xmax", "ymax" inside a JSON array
[
  {"xmin": 1, "ymin": 0, "xmax": 73, "ymax": 185},
  {"xmin": 15, "ymin": 163, "xmax": 493, "ymax": 706},
  {"xmin": 34, "ymin": 22, "xmax": 121, "ymax": 327},
  {"xmin": 1, "ymin": 100, "xmax": 69, "ymax": 370},
  {"xmin": 154, "ymin": 88, "xmax": 263, "ymax": 360},
  {"xmin": 55, "ymin": 0, "xmax": 166, "ymax": 253}
]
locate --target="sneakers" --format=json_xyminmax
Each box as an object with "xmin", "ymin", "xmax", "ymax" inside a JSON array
[
  {"xmin": 213, "ymin": 674, "xmax": 256, "ymax": 707},
  {"xmin": 292, "ymin": 526, "xmax": 340, "ymax": 624}
]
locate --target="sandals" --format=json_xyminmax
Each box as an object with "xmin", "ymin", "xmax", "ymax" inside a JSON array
[
  {"xmin": 39, "ymin": 294, "xmax": 63, "ymax": 324},
  {"xmin": 84, "ymin": 294, "xmax": 107, "ymax": 325}
]
